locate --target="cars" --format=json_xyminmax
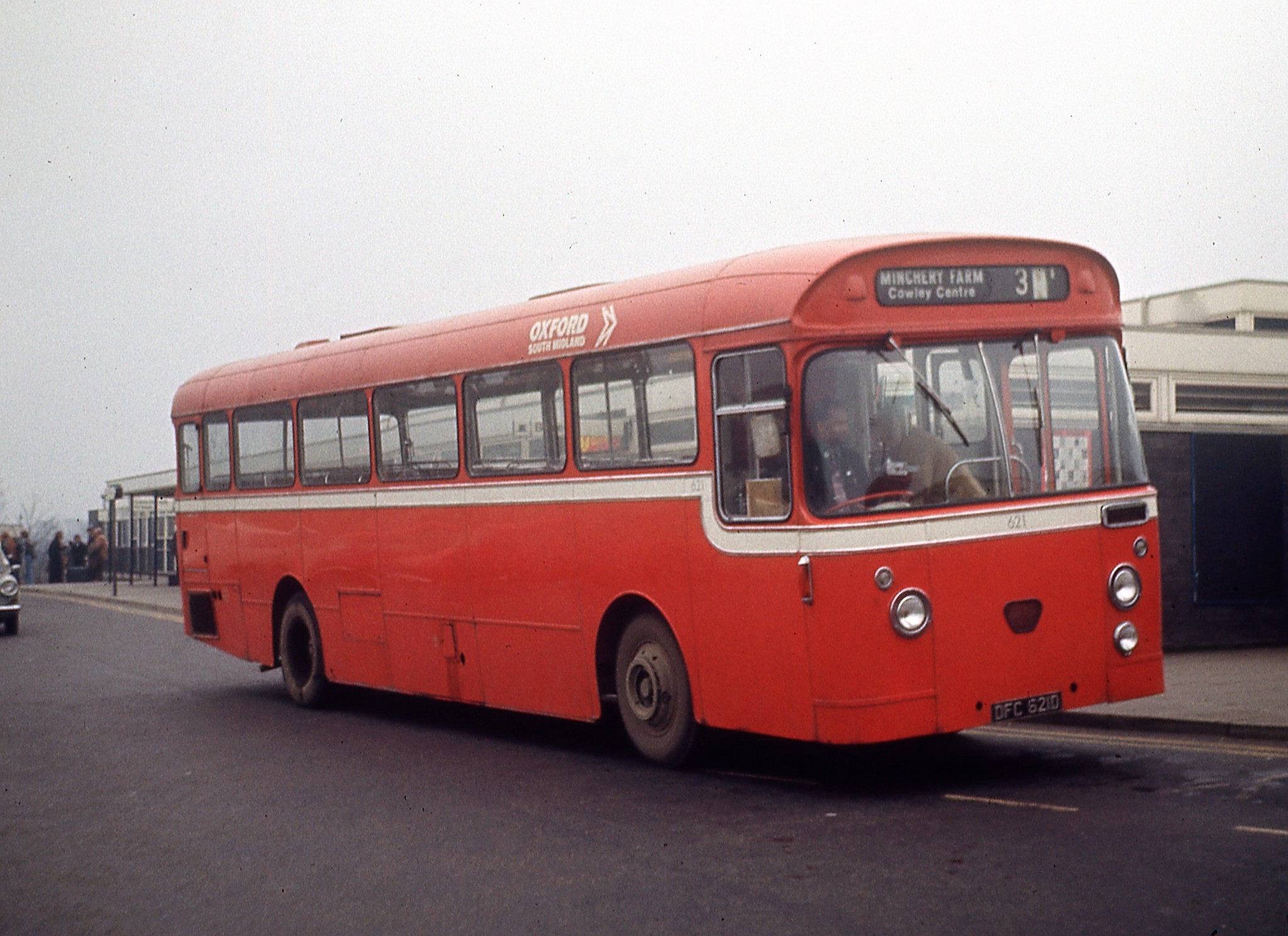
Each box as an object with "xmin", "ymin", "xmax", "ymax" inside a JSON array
[{"xmin": 0, "ymin": 547, "xmax": 21, "ymax": 632}]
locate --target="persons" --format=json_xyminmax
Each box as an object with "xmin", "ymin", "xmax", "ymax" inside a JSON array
[
  {"xmin": 875, "ymin": 403, "xmax": 985, "ymax": 505},
  {"xmin": 804, "ymin": 399, "xmax": 870, "ymax": 515},
  {"xmin": 86, "ymin": 527, "xmax": 108, "ymax": 582},
  {"xmin": 70, "ymin": 536, "xmax": 86, "ymax": 566},
  {"xmin": 19, "ymin": 530, "xmax": 33, "ymax": 584},
  {"xmin": 0, "ymin": 531, "xmax": 19, "ymax": 582},
  {"xmin": 49, "ymin": 532, "xmax": 66, "ymax": 582}
]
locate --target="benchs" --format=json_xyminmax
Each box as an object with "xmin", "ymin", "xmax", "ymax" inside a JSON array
[
  {"xmin": 208, "ymin": 455, "xmax": 621, "ymax": 491},
  {"xmin": 150, "ymin": 568, "xmax": 178, "ymax": 586}
]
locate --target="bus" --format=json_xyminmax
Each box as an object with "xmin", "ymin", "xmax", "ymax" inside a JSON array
[{"xmin": 170, "ymin": 230, "xmax": 1167, "ymax": 768}]
